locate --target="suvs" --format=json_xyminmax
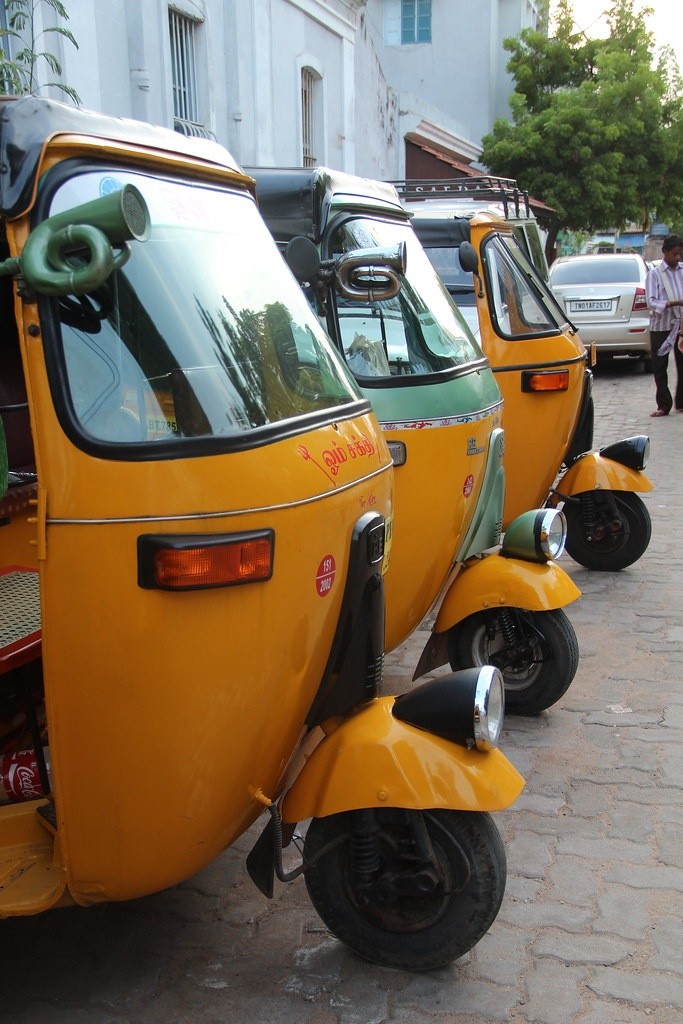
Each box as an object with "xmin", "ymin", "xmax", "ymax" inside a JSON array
[{"xmin": 320, "ymin": 174, "xmax": 562, "ymax": 365}]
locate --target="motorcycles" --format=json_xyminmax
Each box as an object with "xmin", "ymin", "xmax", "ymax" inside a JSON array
[
  {"xmin": 251, "ymin": 171, "xmax": 580, "ymax": 715},
  {"xmin": 0, "ymin": 96, "xmax": 528, "ymax": 972},
  {"xmin": 410, "ymin": 214, "xmax": 653, "ymax": 572}
]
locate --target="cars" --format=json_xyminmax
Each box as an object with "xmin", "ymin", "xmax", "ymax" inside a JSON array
[{"xmin": 547, "ymin": 254, "xmax": 683, "ymax": 373}]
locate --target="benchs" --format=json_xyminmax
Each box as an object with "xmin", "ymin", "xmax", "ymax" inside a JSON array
[{"xmin": 0, "ymin": 330, "xmax": 37, "ymax": 517}]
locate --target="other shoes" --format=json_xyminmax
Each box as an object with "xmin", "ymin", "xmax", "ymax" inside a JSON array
[
  {"xmin": 650, "ymin": 406, "xmax": 666, "ymax": 416},
  {"xmin": 674, "ymin": 403, "xmax": 683, "ymax": 413}
]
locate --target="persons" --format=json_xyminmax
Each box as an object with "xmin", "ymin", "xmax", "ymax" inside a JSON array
[{"xmin": 644, "ymin": 236, "xmax": 683, "ymax": 416}]
